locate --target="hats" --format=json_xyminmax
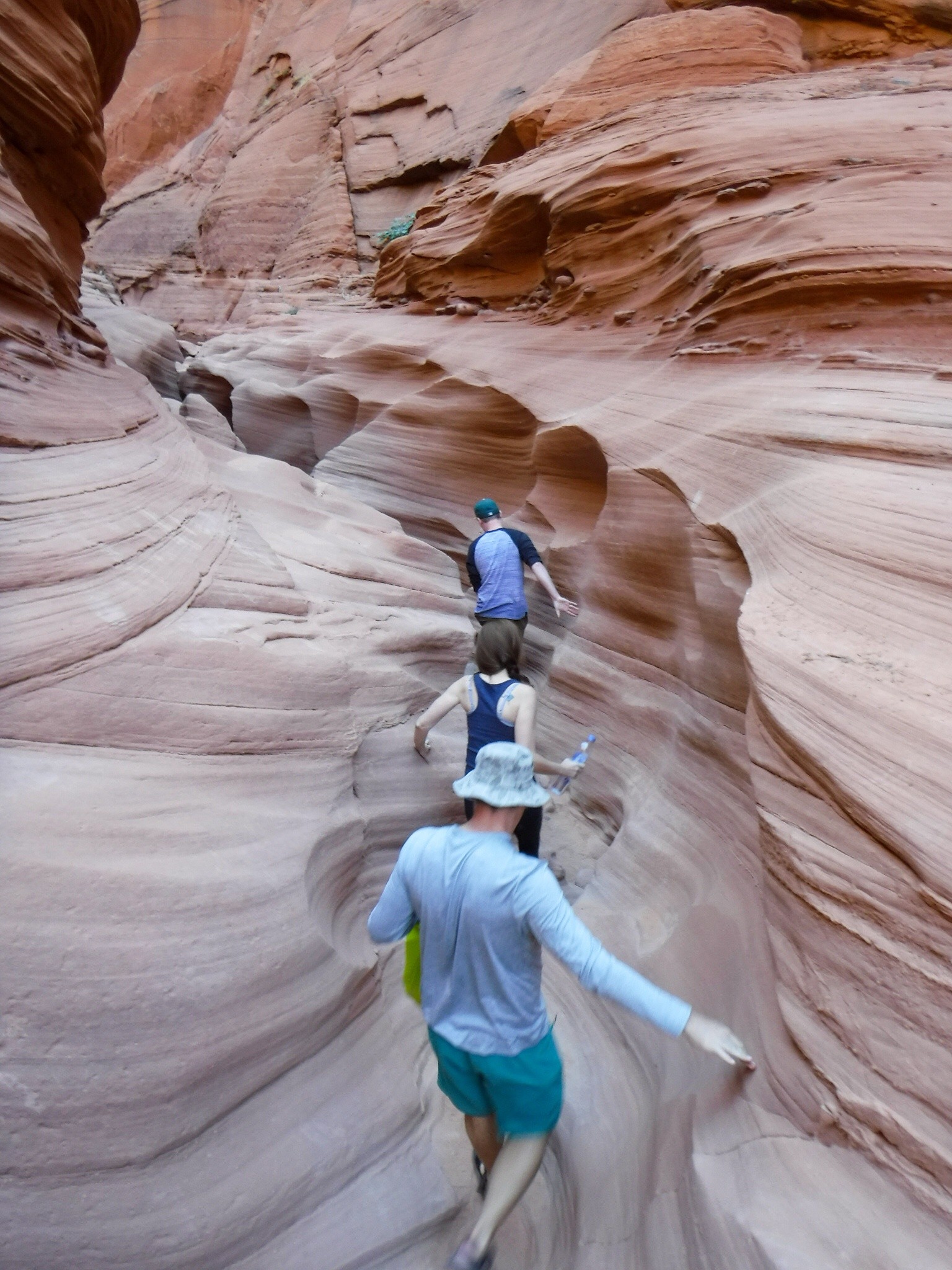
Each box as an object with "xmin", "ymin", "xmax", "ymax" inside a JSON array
[
  {"xmin": 452, "ymin": 740, "xmax": 551, "ymax": 808},
  {"xmin": 474, "ymin": 498, "xmax": 499, "ymax": 519}
]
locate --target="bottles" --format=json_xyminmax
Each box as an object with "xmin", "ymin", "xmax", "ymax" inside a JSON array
[{"xmin": 550, "ymin": 734, "xmax": 596, "ymax": 794}]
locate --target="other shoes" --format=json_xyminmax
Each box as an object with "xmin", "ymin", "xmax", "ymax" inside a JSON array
[
  {"xmin": 446, "ymin": 1237, "xmax": 495, "ymax": 1269},
  {"xmin": 470, "ymin": 1150, "xmax": 492, "ymax": 1203}
]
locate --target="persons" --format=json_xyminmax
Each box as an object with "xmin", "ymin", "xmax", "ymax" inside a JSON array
[
  {"xmin": 412, "ymin": 618, "xmax": 586, "ymax": 858},
  {"xmin": 365, "ymin": 741, "xmax": 758, "ymax": 1270},
  {"xmin": 466, "ymin": 496, "xmax": 579, "ymax": 644}
]
